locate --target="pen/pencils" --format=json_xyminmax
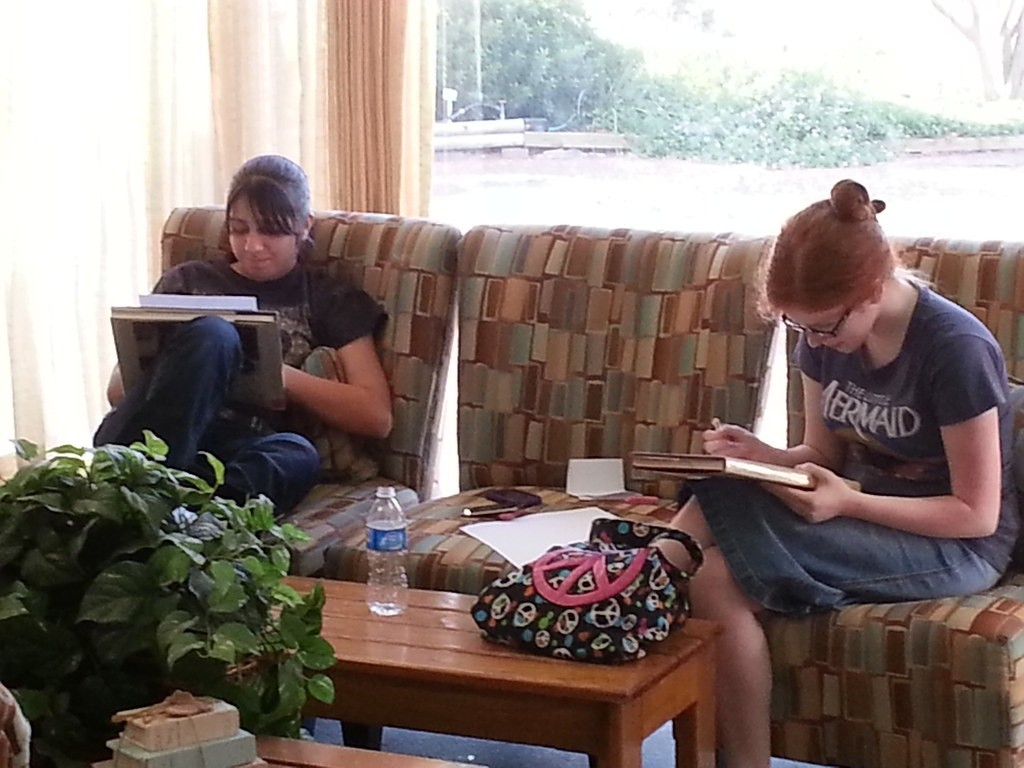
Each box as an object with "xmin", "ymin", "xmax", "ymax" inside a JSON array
[{"xmin": 710, "ymin": 417, "xmax": 731, "ymax": 441}]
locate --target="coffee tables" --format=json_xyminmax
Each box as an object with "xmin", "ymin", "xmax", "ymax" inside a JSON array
[{"xmin": 271, "ymin": 576, "xmax": 723, "ymax": 768}]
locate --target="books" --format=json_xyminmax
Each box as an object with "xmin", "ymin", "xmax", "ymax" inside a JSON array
[
  {"xmin": 632, "ymin": 451, "xmax": 861, "ymax": 493},
  {"xmin": 109, "ymin": 306, "xmax": 287, "ymax": 412}
]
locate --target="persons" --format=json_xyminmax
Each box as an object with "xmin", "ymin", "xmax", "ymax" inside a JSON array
[
  {"xmin": 669, "ymin": 179, "xmax": 1018, "ymax": 768},
  {"xmin": 100, "ymin": 161, "xmax": 393, "ymax": 522}
]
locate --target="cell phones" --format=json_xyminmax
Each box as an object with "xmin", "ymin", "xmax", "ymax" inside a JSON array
[{"xmin": 461, "ymin": 502, "xmax": 519, "ymax": 516}]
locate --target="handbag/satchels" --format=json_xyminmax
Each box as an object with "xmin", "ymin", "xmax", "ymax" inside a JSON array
[{"xmin": 470, "ymin": 519, "xmax": 703, "ymax": 664}]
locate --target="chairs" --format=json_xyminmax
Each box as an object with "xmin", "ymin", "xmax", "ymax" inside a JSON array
[
  {"xmin": 754, "ymin": 238, "xmax": 1024, "ymax": 768},
  {"xmin": 322, "ymin": 225, "xmax": 778, "ymax": 751},
  {"xmin": 161, "ymin": 206, "xmax": 462, "ymax": 580}
]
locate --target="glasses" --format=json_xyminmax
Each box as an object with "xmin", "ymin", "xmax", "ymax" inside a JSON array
[{"xmin": 781, "ymin": 303, "xmax": 853, "ymax": 338}]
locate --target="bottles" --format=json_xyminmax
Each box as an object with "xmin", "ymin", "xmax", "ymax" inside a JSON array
[{"xmin": 365, "ymin": 487, "xmax": 408, "ymax": 615}]
